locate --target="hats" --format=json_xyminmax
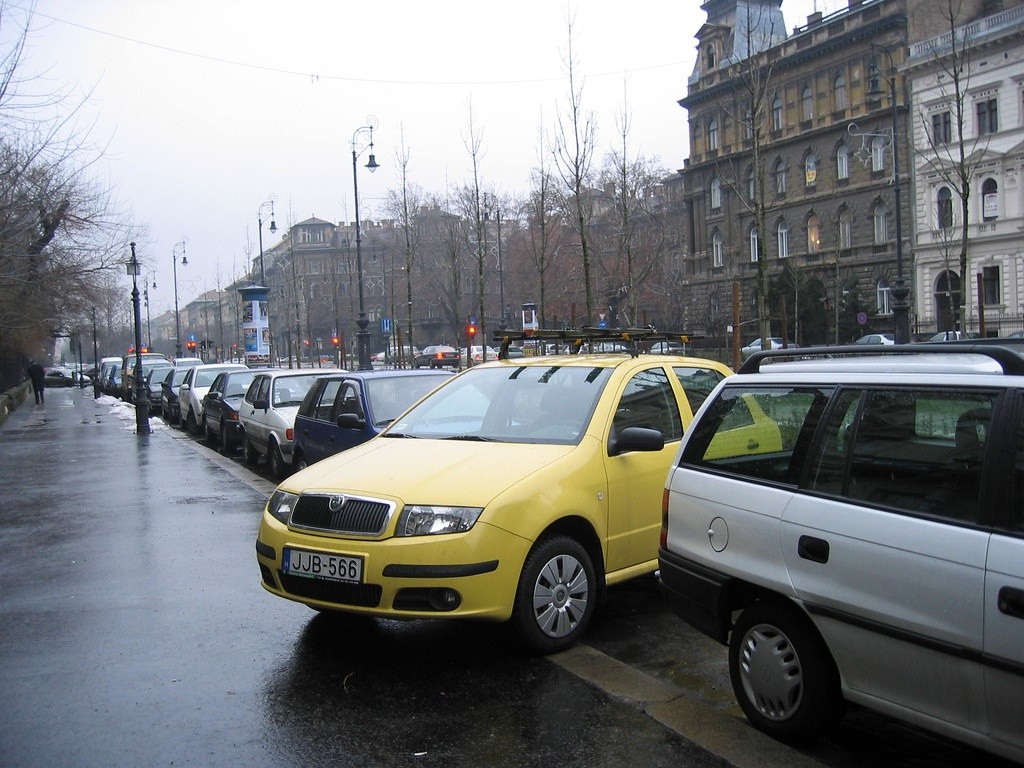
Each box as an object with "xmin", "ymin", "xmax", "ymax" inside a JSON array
[{"xmin": 28, "ymin": 359, "xmax": 34, "ymax": 364}]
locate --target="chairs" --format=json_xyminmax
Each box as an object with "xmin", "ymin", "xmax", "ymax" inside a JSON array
[
  {"xmin": 277, "ymin": 388, "xmax": 291, "ymax": 402},
  {"xmin": 174, "ymin": 374, "xmax": 185, "ymax": 384},
  {"xmin": 196, "ymin": 375, "xmax": 209, "ymax": 386},
  {"xmin": 529, "ymin": 388, "xmax": 582, "ymax": 433},
  {"xmin": 952, "ymin": 409, "xmax": 990, "ymax": 520},
  {"xmin": 228, "ymin": 383, "xmax": 244, "ymax": 396}
]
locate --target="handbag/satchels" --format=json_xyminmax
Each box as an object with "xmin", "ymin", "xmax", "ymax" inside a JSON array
[{"xmin": 39, "ymin": 379, "xmax": 50, "ymax": 388}]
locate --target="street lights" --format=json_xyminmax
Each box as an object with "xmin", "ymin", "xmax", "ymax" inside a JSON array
[
  {"xmin": 173, "ymin": 240, "xmax": 189, "ymax": 358},
  {"xmin": 351, "ymin": 124, "xmax": 381, "ymax": 368},
  {"xmin": 145, "ymin": 269, "xmax": 159, "ymax": 353},
  {"xmin": 258, "ymin": 200, "xmax": 277, "ymax": 368},
  {"xmin": 126, "ymin": 241, "xmax": 152, "ymax": 435}
]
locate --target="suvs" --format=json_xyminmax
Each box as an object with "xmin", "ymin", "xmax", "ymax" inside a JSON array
[{"xmin": 655, "ymin": 338, "xmax": 1024, "ymax": 768}]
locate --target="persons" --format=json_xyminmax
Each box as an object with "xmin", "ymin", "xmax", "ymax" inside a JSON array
[{"xmin": 27, "ymin": 359, "xmax": 45, "ymax": 404}]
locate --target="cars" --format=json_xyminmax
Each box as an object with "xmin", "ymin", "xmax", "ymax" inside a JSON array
[
  {"xmin": 459, "ymin": 345, "xmax": 499, "ymax": 369},
  {"xmin": 178, "ymin": 363, "xmax": 255, "ymax": 435},
  {"xmin": 492, "ymin": 345, "xmax": 525, "ymax": 359},
  {"xmin": 201, "ymin": 366, "xmax": 298, "ymax": 453},
  {"xmin": 413, "ymin": 346, "xmax": 460, "ymax": 370},
  {"xmin": 42, "ymin": 352, "xmax": 208, "ymax": 425},
  {"xmin": 928, "ymin": 331, "xmax": 965, "ymax": 341},
  {"xmin": 237, "ymin": 367, "xmax": 359, "ymax": 478},
  {"xmin": 852, "ymin": 334, "xmax": 895, "ymax": 346},
  {"xmin": 650, "ymin": 341, "xmax": 690, "ymax": 356},
  {"xmin": 544, "ymin": 339, "xmax": 628, "ymax": 356},
  {"xmin": 225, "ymin": 346, "xmax": 423, "ymax": 370},
  {"xmin": 292, "ymin": 367, "xmax": 521, "ymax": 483},
  {"xmin": 253, "ymin": 350, "xmax": 784, "ymax": 655},
  {"xmin": 739, "ymin": 336, "xmax": 800, "ymax": 361}
]
